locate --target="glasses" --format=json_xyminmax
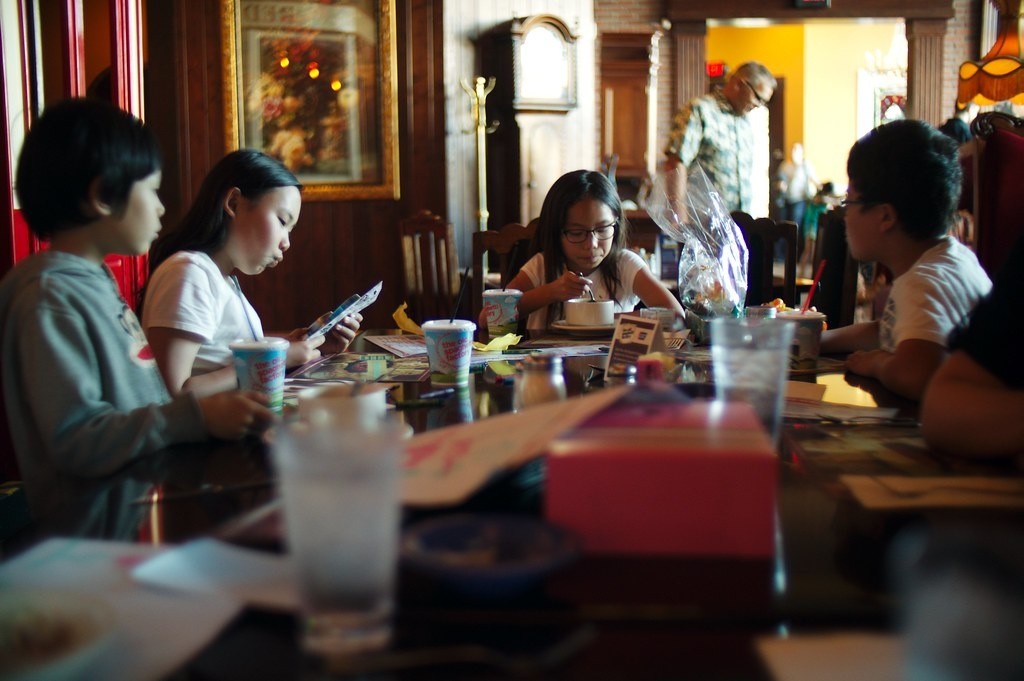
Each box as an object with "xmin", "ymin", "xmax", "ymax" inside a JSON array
[
  {"xmin": 838, "ymin": 195, "xmax": 901, "ymax": 221},
  {"xmin": 561, "ymin": 216, "xmax": 620, "ymax": 244},
  {"xmin": 744, "ymin": 80, "xmax": 769, "ymax": 108}
]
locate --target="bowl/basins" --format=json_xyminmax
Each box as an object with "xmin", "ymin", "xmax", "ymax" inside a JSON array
[{"xmin": 563, "ymin": 298, "xmax": 614, "ymax": 325}]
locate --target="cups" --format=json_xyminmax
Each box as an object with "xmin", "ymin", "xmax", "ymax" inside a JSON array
[
  {"xmin": 711, "ymin": 318, "xmax": 795, "ymax": 446},
  {"xmin": 299, "ymin": 382, "xmax": 387, "ymax": 423},
  {"xmin": 422, "ymin": 320, "xmax": 477, "ymax": 387},
  {"xmin": 230, "ymin": 337, "xmax": 290, "ymax": 418},
  {"xmin": 264, "ymin": 423, "xmax": 414, "ymax": 653},
  {"xmin": 777, "ymin": 311, "xmax": 826, "ymax": 373},
  {"xmin": 482, "ymin": 289, "xmax": 523, "ymax": 339},
  {"xmin": 746, "ymin": 307, "xmax": 776, "ymax": 319}
]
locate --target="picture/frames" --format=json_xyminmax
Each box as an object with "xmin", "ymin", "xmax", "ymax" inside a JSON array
[{"xmin": 221, "ymin": 0, "xmax": 401, "ymax": 202}]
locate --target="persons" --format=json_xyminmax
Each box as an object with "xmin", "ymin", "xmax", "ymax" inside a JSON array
[
  {"xmin": 0, "ymin": 98, "xmax": 283, "ymax": 542},
  {"xmin": 498, "ymin": 170, "xmax": 687, "ymax": 330},
  {"xmin": 141, "ymin": 149, "xmax": 363, "ymax": 402},
  {"xmin": 818, "ymin": 120, "xmax": 994, "ymax": 397},
  {"xmin": 770, "ymin": 143, "xmax": 838, "ymax": 281},
  {"xmin": 666, "ymin": 62, "xmax": 778, "ymax": 243},
  {"xmin": 919, "ymin": 241, "xmax": 1024, "ymax": 464}
]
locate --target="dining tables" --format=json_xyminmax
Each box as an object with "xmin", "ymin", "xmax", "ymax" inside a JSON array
[{"xmin": 0, "ymin": 326, "xmax": 1024, "ymax": 681}]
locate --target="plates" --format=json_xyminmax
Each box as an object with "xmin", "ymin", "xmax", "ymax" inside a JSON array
[{"xmin": 551, "ymin": 319, "xmax": 617, "ymax": 337}]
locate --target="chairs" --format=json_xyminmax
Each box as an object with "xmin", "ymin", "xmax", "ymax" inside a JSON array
[
  {"xmin": 473, "ymin": 217, "xmax": 544, "ymax": 321},
  {"xmin": 812, "ymin": 205, "xmax": 859, "ymax": 330},
  {"xmin": 397, "ymin": 209, "xmax": 454, "ymax": 325},
  {"xmin": 677, "ymin": 210, "xmax": 802, "ymax": 308}
]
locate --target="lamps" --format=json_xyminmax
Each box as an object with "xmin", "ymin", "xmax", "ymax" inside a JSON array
[{"xmin": 956, "ymin": 0, "xmax": 1024, "ymax": 110}]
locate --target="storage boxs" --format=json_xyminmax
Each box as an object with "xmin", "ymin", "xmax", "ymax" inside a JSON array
[{"xmin": 548, "ymin": 400, "xmax": 779, "ymax": 560}]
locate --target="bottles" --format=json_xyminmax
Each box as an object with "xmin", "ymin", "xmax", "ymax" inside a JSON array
[{"xmin": 521, "ymin": 356, "xmax": 566, "ymax": 409}]
